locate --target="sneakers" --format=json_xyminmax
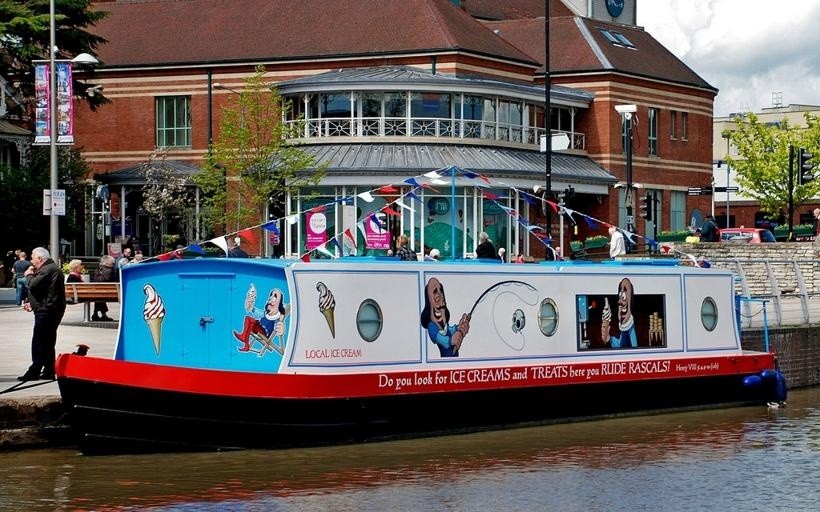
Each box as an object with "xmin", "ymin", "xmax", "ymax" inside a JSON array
[
  {"xmin": 39, "ymin": 373, "xmax": 54, "ymax": 380},
  {"xmin": 100, "ymin": 315, "xmax": 113, "ymax": 321},
  {"xmin": 92, "ymin": 315, "xmax": 100, "ymax": 320},
  {"xmin": 17, "ymin": 374, "xmax": 39, "ymax": 382}
]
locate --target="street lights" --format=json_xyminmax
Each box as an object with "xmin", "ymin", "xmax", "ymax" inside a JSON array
[
  {"xmin": 212, "ymin": 82, "xmax": 245, "ymax": 237},
  {"xmin": 31, "ymin": 52, "xmax": 99, "ymax": 266},
  {"xmin": 722, "ymin": 133, "xmax": 731, "ymax": 228}
]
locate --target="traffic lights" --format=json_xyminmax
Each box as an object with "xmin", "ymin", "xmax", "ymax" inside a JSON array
[
  {"xmin": 798, "ymin": 147, "xmax": 814, "ymax": 183},
  {"xmin": 640, "ymin": 192, "xmax": 652, "ymax": 221}
]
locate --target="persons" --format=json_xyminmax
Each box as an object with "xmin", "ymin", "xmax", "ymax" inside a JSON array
[
  {"xmin": 429, "ymin": 249, "xmax": 441, "ymax": 259},
  {"xmin": 94, "ymin": 247, "xmax": 143, "ymax": 282},
  {"xmin": 66, "ymin": 259, "xmax": 114, "ymax": 322},
  {"xmin": 0, "ymin": 249, "xmax": 34, "ymax": 305},
  {"xmin": 476, "ymin": 232, "xmax": 496, "ymax": 260},
  {"xmin": 424, "ymin": 244, "xmax": 432, "ymax": 256},
  {"xmin": 697, "ymin": 256, "xmax": 712, "ymax": 268},
  {"xmin": 544, "ymin": 242, "xmax": 560, "ymax": 261},
  {"xmin": 173, "ymin": 245, "xmax": 183, "ymax": 259},
  {"xmin": 515, "ymin": 255, "xmax": 525, "ymax": 263},
  {"xmin": 396, "ymin": 235, "xmax": 419, "ymax": 261},
  {"xmin": 217, "ymin": 237, "xmax": 249, "ymax": 258},
  {"xmin": 18, "ymin": 245, "xmax": 66, "ymax": 383},
  {"xmin": 694, "ymin": 214, "xmax": 718, "ymax": 242},
  {"xmin": 608, "ymin": 226, "xmax": 626, "ymax": 260},
  {"xmin": 496, "ymin": 247, "xmax": 506, "ymax": 263},
  {"xmin": 813, "ymin": 208, "xmax": 820, "ymax": 236}
]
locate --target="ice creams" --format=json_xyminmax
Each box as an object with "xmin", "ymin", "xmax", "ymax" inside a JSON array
[
  {"xmin": 144, "ymin": 284, "xmax": 165, "ymax": 355},
  {"xmin": 247, "ymin": 285, "xmax": 256, "ymax": 309},
  {"xmin": 316, "ymin": 282, "xmax": 336, "ymax": 339},
  {"xmin": 602, "ymin": 298, "xmax": 611, "ymax": 341}
]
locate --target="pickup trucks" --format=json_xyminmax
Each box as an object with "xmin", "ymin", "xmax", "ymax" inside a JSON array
[{"xmin": 720, "ymin": 225, "xmax": 777, "ymax": 243}]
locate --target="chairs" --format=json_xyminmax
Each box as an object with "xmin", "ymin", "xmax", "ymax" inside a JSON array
[{"xmin": 249, "ymin": 305, "xmax": 291, "ymax": 357}]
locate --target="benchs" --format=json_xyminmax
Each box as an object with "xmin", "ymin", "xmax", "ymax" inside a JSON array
[{"xmin": 65, "ymin": 283, "xmax": 120, "ymax": 323}]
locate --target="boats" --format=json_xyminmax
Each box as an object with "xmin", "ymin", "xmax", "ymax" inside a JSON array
[{"xmin": 52, "ymin": 166, "xmax": 785, "ymax": 455}]
